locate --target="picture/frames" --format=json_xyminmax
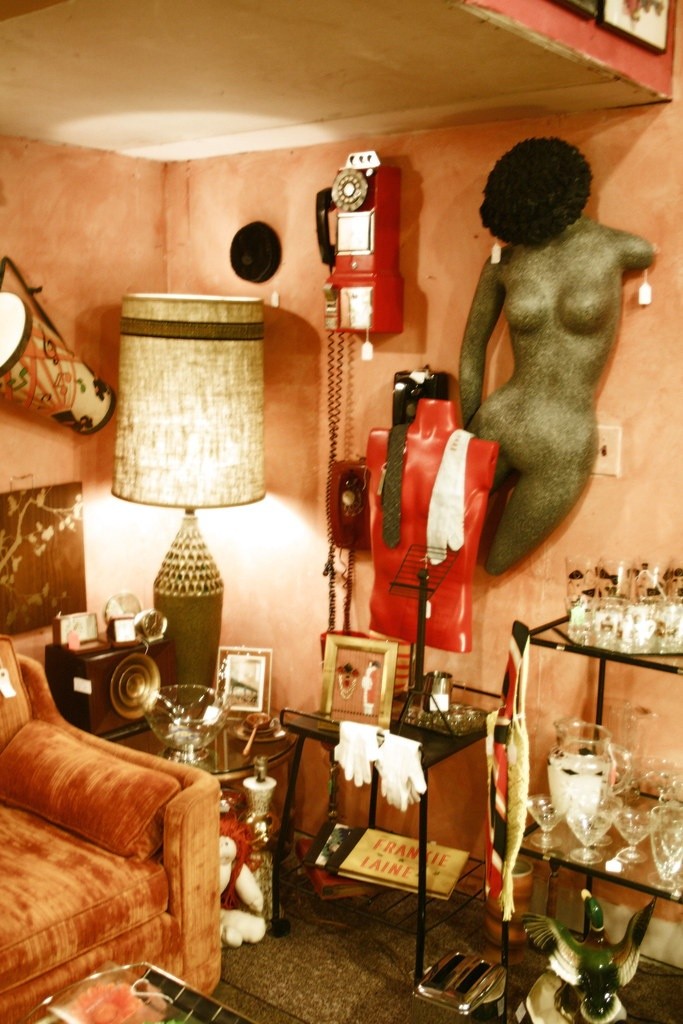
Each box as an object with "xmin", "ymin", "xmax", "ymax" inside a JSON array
[
  {"xmin": 213, "ymin": 648, "xmax": 273, "ymax": 720},
  {"xmin": 552, "ymin": 0, "xmax": 671, "ymax": 55},
  {"xmin": 317, "ymin": 634, "xmax": 399, "ymax": 733}
]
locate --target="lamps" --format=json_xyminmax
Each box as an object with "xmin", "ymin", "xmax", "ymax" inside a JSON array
[{"xmin": 110, "ymin": 292, "xmax": 265, "ymax": 708}]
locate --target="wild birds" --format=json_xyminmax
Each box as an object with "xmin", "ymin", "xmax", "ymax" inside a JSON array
[{"xmin": 521, "ymin": 888, "xmax": 657, "ymax": 1024}]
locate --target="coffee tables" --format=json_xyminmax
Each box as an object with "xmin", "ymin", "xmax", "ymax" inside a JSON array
[{"xmin": 20, "ymin": 963, "xmax": 257, "ymax": 1024}]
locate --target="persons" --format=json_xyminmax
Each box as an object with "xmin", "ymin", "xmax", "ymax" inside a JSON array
[{"xmin": 452, "ymin": 135, "xmax": 655, "ymax": 578}]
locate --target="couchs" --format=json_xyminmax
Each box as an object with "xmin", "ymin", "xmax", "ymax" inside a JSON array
[{"xmin": 0, "ymin": 635, "xmax": 222, "ymax": 1024}]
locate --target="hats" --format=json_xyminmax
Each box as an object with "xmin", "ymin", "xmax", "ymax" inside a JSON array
[{"xmin": 480, "ymin": 137, "xmax": 592, "ymax": 254}]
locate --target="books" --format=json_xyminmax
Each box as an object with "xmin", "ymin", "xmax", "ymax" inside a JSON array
[{"xmin": 316, "ymin": 824, "xmax": 469, "ymax": 900}]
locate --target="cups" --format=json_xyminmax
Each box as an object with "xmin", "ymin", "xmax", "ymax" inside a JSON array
[{"xmin": 422, "ymin": 670, "xmax": 453, "ymax": 714}]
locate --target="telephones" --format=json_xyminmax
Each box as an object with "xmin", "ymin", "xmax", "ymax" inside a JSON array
[
  {"xmin": 329, "ymin": 461, "xmax": 372, "ymax": 550},
  {"xmin": 318, "ymin": 150, "xmax": 403, "ymax": 333}
]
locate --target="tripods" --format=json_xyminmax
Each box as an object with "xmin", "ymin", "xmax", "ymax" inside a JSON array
[{"xmin": 386, "ymin": 544, "xmax": 457, "ymax": 739}]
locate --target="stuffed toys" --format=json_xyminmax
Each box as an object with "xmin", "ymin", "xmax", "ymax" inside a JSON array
[{"xmin": 219, "ymin": 822, "xmax": 267, "ymax": 947}]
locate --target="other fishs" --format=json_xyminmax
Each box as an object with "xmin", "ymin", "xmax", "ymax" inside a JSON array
[{"xmin": 498, "ymin": 712, "xmax": 531, "ymax": 921}]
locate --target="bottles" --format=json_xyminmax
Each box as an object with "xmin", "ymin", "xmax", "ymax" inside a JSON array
[{"xmin": 547, "ymin": 720, "xmax": 616, "ymax": 808}]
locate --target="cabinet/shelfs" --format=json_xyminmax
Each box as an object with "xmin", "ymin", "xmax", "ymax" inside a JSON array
[{"xmin": 489, "ymin": 613, "xmax": 683, "ymax": 1024}]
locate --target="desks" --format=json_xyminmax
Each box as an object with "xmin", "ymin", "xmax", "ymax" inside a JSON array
[
  {"xmin": 108, "ymin": 705, "xmax": 299, "ymax": 862},
  {"xmin": 269, "ymin": 684, "xmax": 500, "ymax": 989}
]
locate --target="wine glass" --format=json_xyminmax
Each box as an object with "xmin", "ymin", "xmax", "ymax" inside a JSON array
[
  {"xmin": 527, "ymin": 745, "xmax": 683, "ymax": 894},
  {"xmin": 143, "ymin": 684, "xmax": 230, "ymax": 768}
]
